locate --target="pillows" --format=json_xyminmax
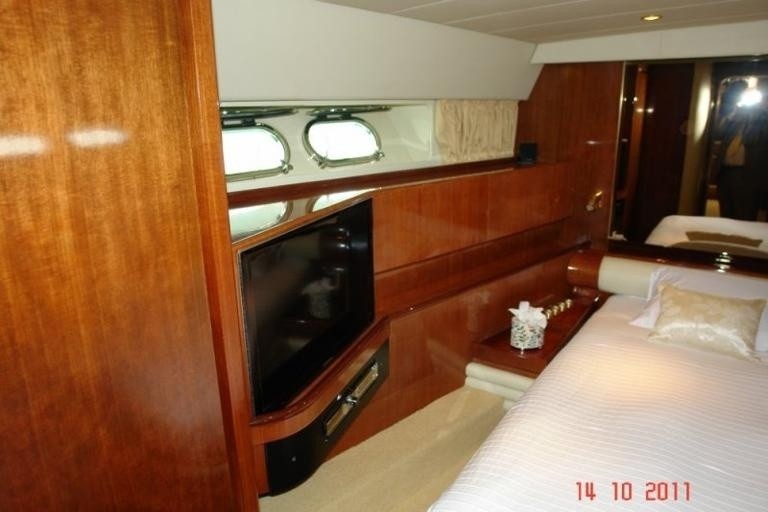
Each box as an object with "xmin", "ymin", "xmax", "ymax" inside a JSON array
[
  {"xmin": 628, "ymin": 265, "xmax": 767, "ymax": 362},
  {"xmin": 666, "ymin": 230, "xmax": 768, "ymax": 260}
]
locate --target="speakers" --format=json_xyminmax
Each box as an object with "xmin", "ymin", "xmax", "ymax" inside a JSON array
[{"xmin": 517, "ymin": 142, "xmax": 538, "ymax": 165}]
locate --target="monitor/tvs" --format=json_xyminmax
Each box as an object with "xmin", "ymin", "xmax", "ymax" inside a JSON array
[{"xmin": 237, "ymin": 196, "xmax": 377, "ymax": 417}]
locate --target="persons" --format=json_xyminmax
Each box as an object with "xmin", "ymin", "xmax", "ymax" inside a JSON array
[{"xmin": 712, "ymin": 80, "xmax": 767, "ymax": 221}]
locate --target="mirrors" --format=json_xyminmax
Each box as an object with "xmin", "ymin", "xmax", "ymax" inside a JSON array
[{"xmin": 606, "ymin": 57, "xmax": 768, "ymax": 261}]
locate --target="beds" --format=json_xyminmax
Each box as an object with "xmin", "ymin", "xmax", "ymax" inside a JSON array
[
  {"xmin": 644, "ymin": 207, "xmax": 768, "ymax": 262},
  {"xmin": 424, "ymin": 255, "xmax": 768, "ymax": 512}
]
